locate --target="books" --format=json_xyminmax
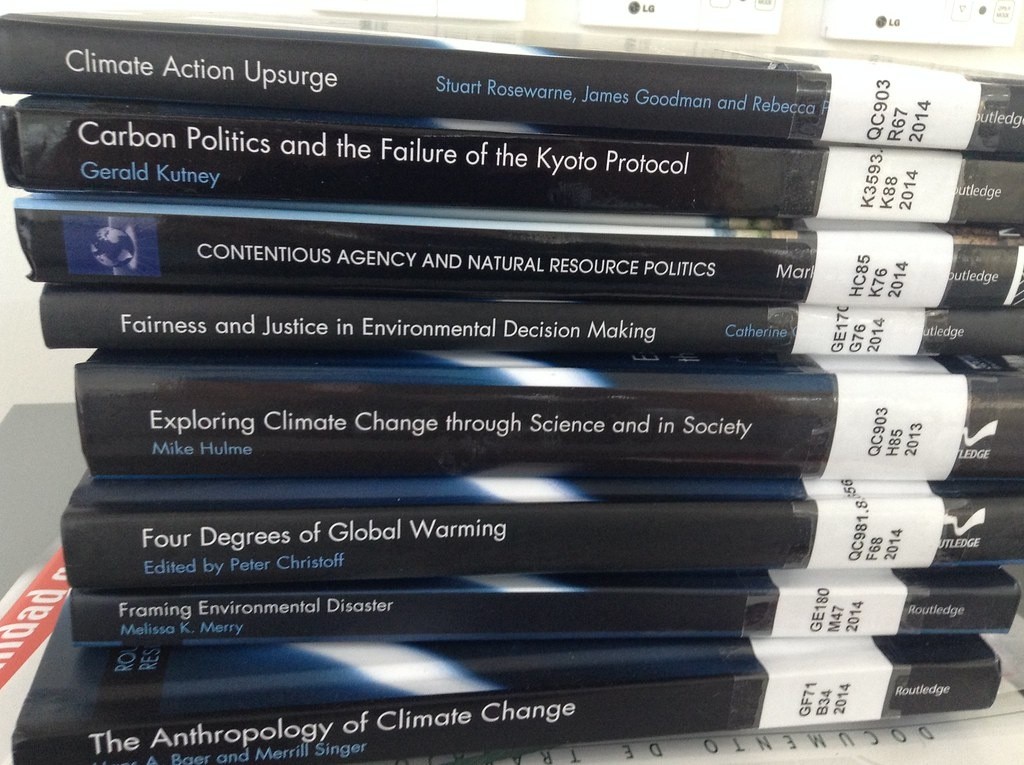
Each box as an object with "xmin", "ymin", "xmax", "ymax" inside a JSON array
[
  {"xmin": 0, "ymin": 106, "xmax": 1024, "ymax": 765},
  {"xmin": 0, "ymin": 8, "xmax": 1024, "ymax": 161}
]
